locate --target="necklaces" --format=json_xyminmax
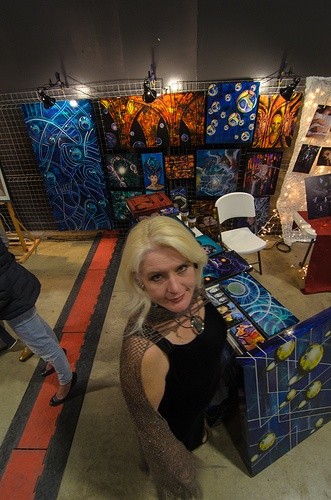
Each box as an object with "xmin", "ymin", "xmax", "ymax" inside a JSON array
[{"xmin": 173, "ymin": 309, "xmax": 205, "ymax": 335}]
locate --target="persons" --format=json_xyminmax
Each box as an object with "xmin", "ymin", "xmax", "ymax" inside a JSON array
[
  {"xmin": 0, "ymin": 230, "xmax": 78, "ymax": 408},
  {"xmin": 119, "ymin": 216, "xmax": 228, "ymax": 500}
]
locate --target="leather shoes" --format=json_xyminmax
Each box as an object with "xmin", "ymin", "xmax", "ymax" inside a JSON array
[{"xmin": 20, "ymin": 347, "xmax": 34, "ymax": 361}]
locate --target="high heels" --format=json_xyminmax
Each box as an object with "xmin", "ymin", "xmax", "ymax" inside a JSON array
[
  {"xmin": 49, "ymin": 372, "xmax": 78, "ymax": 406},
  {"xmin": 40, "ymin": 348, "xmax": 67, "ymax": 377}
]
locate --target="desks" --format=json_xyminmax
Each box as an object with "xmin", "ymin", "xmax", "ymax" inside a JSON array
[{"xmin": 291, "ymin": 211, "xmax": 331, "ymax": 269}]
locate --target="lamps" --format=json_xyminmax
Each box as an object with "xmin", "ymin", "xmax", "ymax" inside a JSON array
[
  {"xmin": 142, "ymin": 83, "xmax": 157, "ymax": 103},
  {"xmin": 279, "ymin": 79, "xmax": 301, "ymax": 101},
  {"xmin": 36, "ymin": 88, "xmax": 56, "ymax": 109}
]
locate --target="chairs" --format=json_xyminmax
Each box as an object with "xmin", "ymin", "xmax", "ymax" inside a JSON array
[{"xmin": 214, "ymin": 192, "xmax": 267, "ymax": 276}]
